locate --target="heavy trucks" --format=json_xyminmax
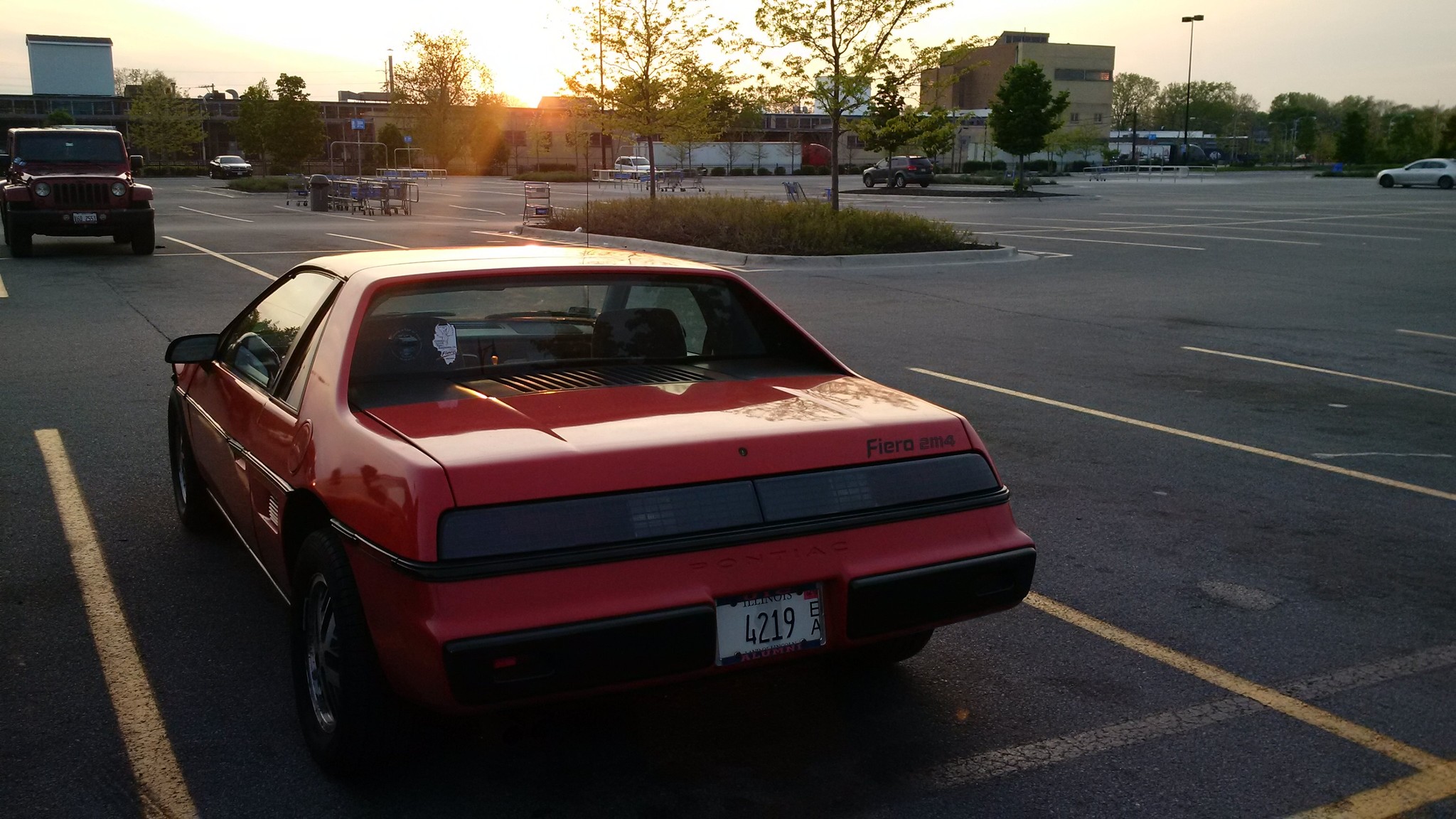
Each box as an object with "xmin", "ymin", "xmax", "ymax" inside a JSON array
[
  {"xmin": 633, "ymin": 141, "xmax": 832, "ymax": 174},
  {"xmin": 1107, "ymin": 143, "xmax": 1212, "ymax": 169}
]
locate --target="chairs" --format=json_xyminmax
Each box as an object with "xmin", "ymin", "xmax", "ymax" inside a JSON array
[
  {"xmin": 590, "ymin": 307, "xmax": 687, "ymax": 359},
  {"xmin": 349, "ymin": 314, "xmax": 465, "ymax": 388}
]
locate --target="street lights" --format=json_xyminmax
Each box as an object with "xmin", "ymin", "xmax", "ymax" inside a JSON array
[
  {"xmin": 1182, "ymin": 15, "xmax": 1204, "ymax": 143},
  {"xmin": 198, "ymin": 95, "xmax": 212, "ymax": 160},
  {"xmin": 1293, "ymin": 117, "xmax": 1317, "ymax": 164},
  {"xmin": 959, "ymin": 112, "xmax": 987, "ymax": 161},
  {"xmin": 1269, "ymin": 122, "xmax": 1287, "ymax": 164},
  {"xmin": 587, "ymin": 105, "xmax": 614, "ymax": 168},
  {"xmin": 1190, "ymin": 117, "xmax": 1219, "ymax": 167}
]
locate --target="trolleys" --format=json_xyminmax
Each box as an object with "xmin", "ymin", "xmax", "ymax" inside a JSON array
[
  {"xmin": 782, "ymin": 182, "xmax": 831, "ymax": 204},
  {"xmin": 1089, "ymin": 164, "xmax": 1109, "ymax": 182},
  {"xmin": 522, "ymin": 182, "xmax": 553, "ymax": 225},
  {"xmin": 1004, "ymin": 170, "xmax": 1039, "ymax": 191},
  {"xmin": 325, "ymin": 174, "xmax": 409, "ymax": 216},
  {"xmin": 286, "ymin": 174, "xmax": 310, "ymax": 206},
  {"xmin": 640, "ymin": 169, "xmax": 707, "ymax": 192}
]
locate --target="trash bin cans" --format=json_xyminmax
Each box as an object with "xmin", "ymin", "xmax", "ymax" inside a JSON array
[{"xmin": 309, "ymin": 174, "xmax": 330, "ymax": 212}]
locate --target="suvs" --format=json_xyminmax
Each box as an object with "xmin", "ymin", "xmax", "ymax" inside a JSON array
[
  {"xmin": 1296, "ymin": 154, "xmax": 1312, "ymax": 160},
  {"xmin": 614, "ymin": 156, "xmax": 656, "ymax": 179},
  {"xmin": 862, "ymin": 154, "xmax": 935, "ymax": 187},
  {"xmin": 0, "ymin": 124, "xmax": 156, "ymax": 259}
]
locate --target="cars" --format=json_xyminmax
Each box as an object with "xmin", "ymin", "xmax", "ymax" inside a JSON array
[
  {"xmin": 1377, "ymin": 158, "xmax": 1456, "ymax": 190},
  {"xmin": 163, "ymin": 247, "xmax": 1040, "ymax": 769},
  {"xmin": 209, "ymin": 155, "xmax": 252, "ymax": 179}
]
